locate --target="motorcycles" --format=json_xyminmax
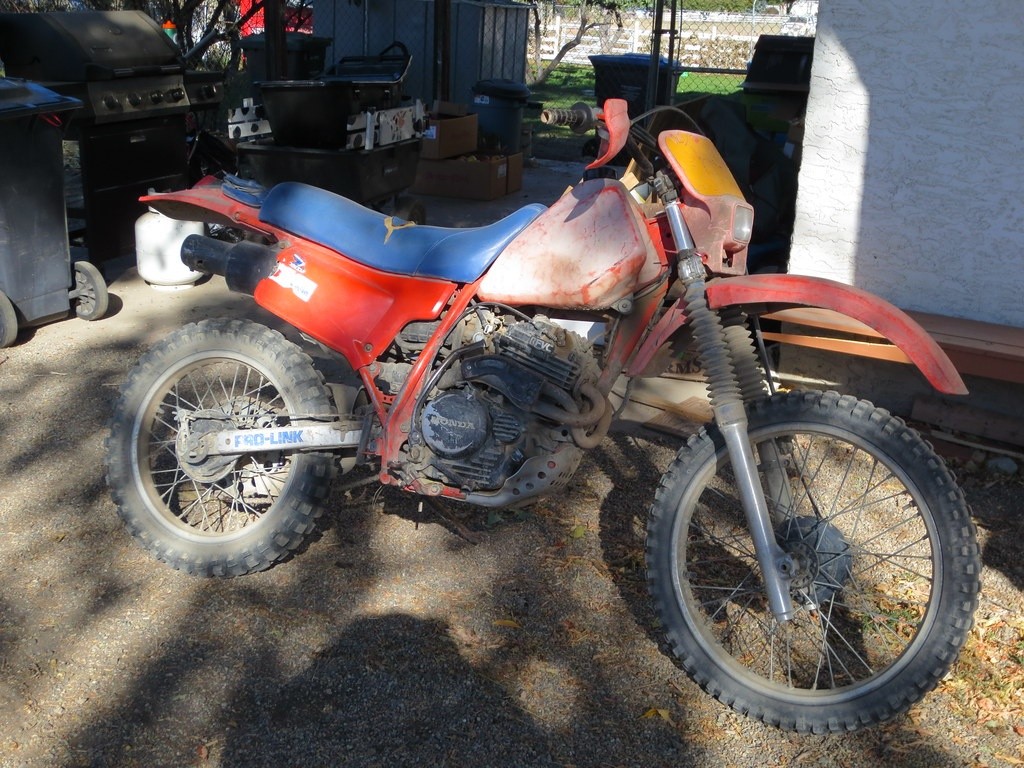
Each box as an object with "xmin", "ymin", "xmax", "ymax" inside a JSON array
[{"xmin": 103, "ymin": 101, "xmax": 984, "ymax": 735}]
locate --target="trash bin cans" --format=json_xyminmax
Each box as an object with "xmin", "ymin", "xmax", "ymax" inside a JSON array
[
  {"xmin": 239, "ymin": 30, "xmax": 332, "ymax": 117},
  {"xmin": 0, "ymin": 78, "xmax": 109, "ymax": 349},
  {"xmin": 475, "ymin": 77, "xmax": 530, "ymax": 165},
  {"xmin": 588, "ymin": 53, "xmax": 682, "ymax": 137}
]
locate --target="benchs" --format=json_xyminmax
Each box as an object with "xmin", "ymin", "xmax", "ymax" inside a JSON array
[{"xmin": 756, "ymin": 305, "xmax": 1023, "ymax": 388}]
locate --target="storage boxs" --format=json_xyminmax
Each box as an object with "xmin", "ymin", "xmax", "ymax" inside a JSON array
[
  {"xmin": 257, "ymin": 54, "xmax": 413, "ymax": 145},
  {"xmin": 235, "ymin": 133, "xmax": 423, "ymax": 204},
  {"xmin": 419, "ymin": 112, "xmax": 478, "ymax": 161},
  {"xmin": 409, "ymin": 157, "xmax": 507, "ymax": 200},
  {"xmin": 508, "ymin": 152, "xmax": 523, "ymax": 194}
]
